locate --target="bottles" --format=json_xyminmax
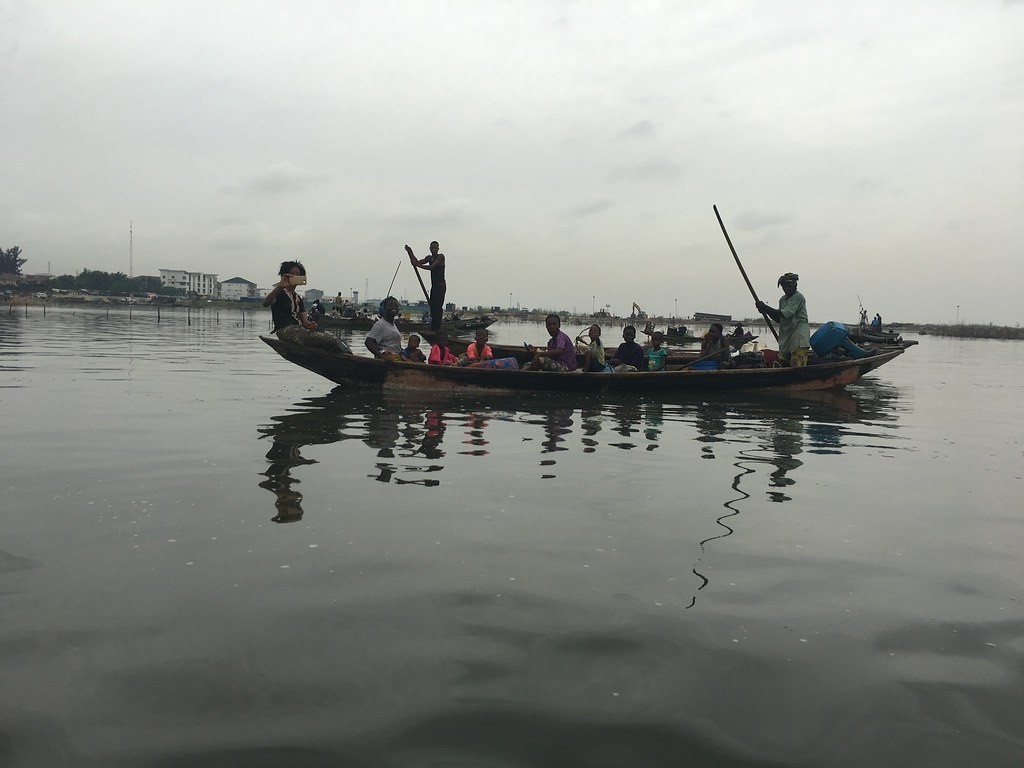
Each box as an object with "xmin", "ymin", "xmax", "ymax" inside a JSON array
[{"xmin": 445, "ymin": 353, "xmax": 482, "ymax": 365}]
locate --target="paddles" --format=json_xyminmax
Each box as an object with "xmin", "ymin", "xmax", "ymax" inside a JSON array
[
  {"xmin": 712, "ymin": 204, "xmax": 780, "ymax": 345},
  {"xmin": 404, "ymin": 245, "xmax": 433, "ymax": 308}
]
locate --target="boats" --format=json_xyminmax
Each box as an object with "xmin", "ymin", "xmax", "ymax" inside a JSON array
[
  {"xmin": 309, "ymin": 309, "xmax": 497, "ymax": 330},
  {"xmin": 258, "ymin": 331, "xmax": 906, "ymax": 394},
  {"xmin": 844, "ymin": 324, "xmax": 900, "ymax": 342},
  {"xmin": 918, "ymin": 332, "xmax": 928, "ymax": 335}
]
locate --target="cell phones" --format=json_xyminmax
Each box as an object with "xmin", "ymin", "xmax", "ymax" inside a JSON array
[{"xmin": 288, "ymin": 276, "xmax": 307, "ymax": 284}]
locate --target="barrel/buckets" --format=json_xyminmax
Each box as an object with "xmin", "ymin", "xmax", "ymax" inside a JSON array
[{"xmin": 690, "ymin": 359, "xmax": 718, "ymax": 370}]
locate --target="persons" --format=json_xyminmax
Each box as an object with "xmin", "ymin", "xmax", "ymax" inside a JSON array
[
  {"xmin": 262, "ymin": 258, "xmax": 352, "ymax": 354},
  {"xmin": 527, "ymin": 314, "xmax": 577, "ymax": 372},
  {"xmin": 754, "ymin": 272, "xmax": 811, "ymax": 368},
  {"xmin": 428, "ymin": 330, "xmax": 454, "ymax": 366},
  {"xmin": 400, "ymin": 335, "xmax": 426, "ymax": 364},
  {"xmin": 610, "ymin": 325, "xmax": 644, "ymax": 372},
  {"xmin": 732, "ymin": 322, "xmax": 744, "ymax": 337},
  {"xmin": 312, "ymin": 291, "xmax": 461, "ymax": 324},
  {"xmin": 858, "ymin": 308, "xmax": 882, "ymax": 331},
  {"xmin": 452, "ymin": 329, "xmax": 493, "ymax": 368},
  {"xmin": 576, "ymin": 324, "xmax": 605, "ymax": 375},
  {"xmin": 405, "ymin": 241, "xmax": 446, "ymax": 330},
  {"xmin": 643, "ymin": 331, "xmax": 667, "ymax": 371},
  {"xmin": 699, "ymin": 322, "xmax": 730, "ymax": 369},
  {"xmin": 365, "ymin": 296, "xmax": 403, "ymax": 361}
]
